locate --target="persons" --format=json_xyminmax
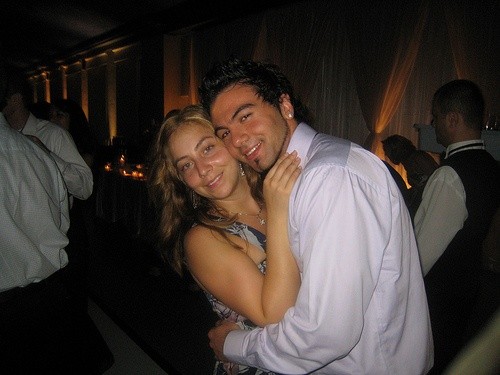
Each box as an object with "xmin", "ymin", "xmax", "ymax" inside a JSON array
[
  {"xmin": 439, "ymin": 310, "xmax": 500, "ymax": 375},
  {"xmin": 412, "ymin": 80, "xmax": 499, "ymax": 375},
  {"xmin": 0, "ymin": 112, "xmax": 70, "ymax": 375},
  {"xmin": 382, "ymin": 134, "xmax": 441, "ymax": 218},
  {"xmin": 147, "ymin": 105, "xmax": 301, "ymax": 374},
  {"xmin": 2, "ymin": 92, "xmax": 93, "ymax": 199},
  {"xmin": 198, "ymin": 60, "xmax": 435, "ymax": 375}
]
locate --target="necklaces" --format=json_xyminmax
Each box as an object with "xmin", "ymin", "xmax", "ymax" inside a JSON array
[{"xmin": 211, "ymin": 207, "xmax": 266, "ymax": 226}]
